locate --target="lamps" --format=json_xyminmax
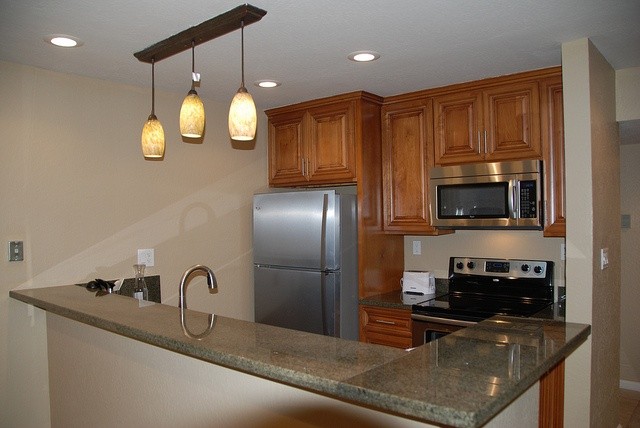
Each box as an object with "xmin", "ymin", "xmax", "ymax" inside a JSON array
[
  {"xmin": 141, "ymin": 58, "xmax": 167, "ymax": 164},
  {"xmin": 227, "ymin": 21, "xmax": 259, "ymax": 143},
  {"xmin": 178, "ymin": 43, "xmax": 207, "ymax": 140}
]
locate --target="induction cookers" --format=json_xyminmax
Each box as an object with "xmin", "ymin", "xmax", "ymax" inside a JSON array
[{"xmin": 413, "ymin": 286, "xmax": 553, "ymax": 321}]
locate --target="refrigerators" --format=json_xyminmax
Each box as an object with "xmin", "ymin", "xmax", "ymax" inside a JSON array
[{"xmin": 253, "ymin": 190, "xmax": 359, "ymax": 339}]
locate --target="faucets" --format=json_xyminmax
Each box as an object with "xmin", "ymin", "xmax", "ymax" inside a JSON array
[{"xmin": 175, "ymin": 263, "xmax": 220, "ymax": 309}]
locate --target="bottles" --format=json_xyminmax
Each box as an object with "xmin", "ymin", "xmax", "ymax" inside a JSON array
[{"xmin": 133, "ymin": 264, "xmax": 148, "ymax": 302}]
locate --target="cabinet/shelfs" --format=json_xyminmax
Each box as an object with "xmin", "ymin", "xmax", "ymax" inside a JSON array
[
  {"xmin": 537, "ymin": 74, "xmax": 566, "ymax": 238},
  {"xmin": 263, "ymin": 90, "xmax": 378, "ymax": 187},
  {"xmin": 432, "ymin": 81, "xmax": 543, "ymax": 166},
  {"xmin": 379, "ymin": 96, "xmax": 457, "ymax": 237},
  {"xmin": 358, "ymin": 304, "xmax": 414, "ymax": 350}
]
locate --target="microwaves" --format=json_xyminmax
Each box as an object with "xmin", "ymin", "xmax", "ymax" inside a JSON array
[{"xmin": 429, "ymin": 159, "xmax": 542, "ymax": 230}]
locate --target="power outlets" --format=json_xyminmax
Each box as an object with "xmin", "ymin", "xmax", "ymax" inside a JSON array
[
  {"xmin": 411, "ymin": 240, "xmax": 422, "ymax": 256},
  {"xmin": 137, "ymin": 247, "xmax": 155, "ymax": 268}
]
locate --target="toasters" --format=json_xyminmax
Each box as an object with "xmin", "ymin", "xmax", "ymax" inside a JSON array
[{"xmin": 399, "ymin": 269, "xmax": 436, "ymax": 295}]
